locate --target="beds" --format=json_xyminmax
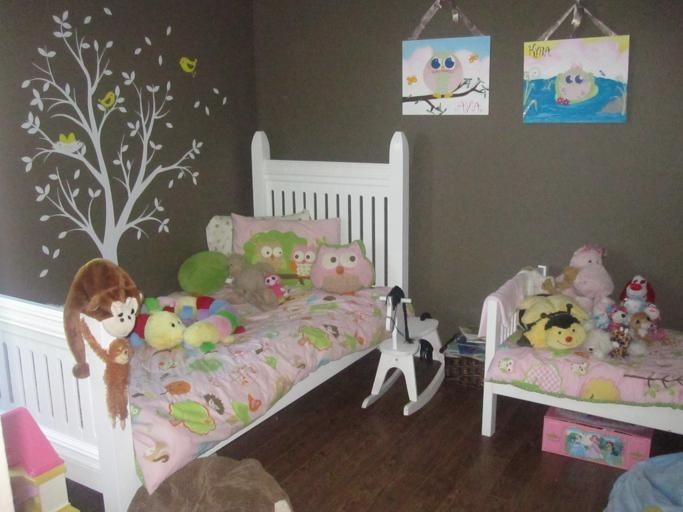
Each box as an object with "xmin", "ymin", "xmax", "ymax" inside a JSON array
[
  {"xmin": 0, "ymin": 130, "xmax": 410, "ymax": 512},
  {"xmin": 480, "ymin": 264, "xmax": 683, "ymax": 437}
]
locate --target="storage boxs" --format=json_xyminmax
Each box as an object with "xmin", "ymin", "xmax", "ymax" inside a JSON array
[{"xmin": 537, "ymin": 404, "xmax": 654, "ymax": 468}]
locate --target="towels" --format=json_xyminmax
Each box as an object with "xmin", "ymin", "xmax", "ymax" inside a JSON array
[{"xmin": 480, "ymin": 264, "xmax": 544, "ymax": 336}]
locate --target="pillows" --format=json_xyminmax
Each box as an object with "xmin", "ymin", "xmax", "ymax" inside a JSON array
[{"xmin": 204, "ymin": 207, "xmax": 343, "ymax": 276}]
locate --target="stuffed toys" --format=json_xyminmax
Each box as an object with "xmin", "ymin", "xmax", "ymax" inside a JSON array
[
  {"xmin": 128, "ymin": 244, "xmax": 290, "ymax": 350},
  {"xmin": 309, "ymin": 241, "xmax": 374, "ymax": 297},
  {"xmin": 79, "ymin": 314, "xmax": 134, "ymax": 430},
  {"xmin": 518, "ymin": 243, "xmax": 671, "ymax": 363},
  {"xmin": 63, "ymin": 259, "xmax": 145, "ymax": 379}
]
